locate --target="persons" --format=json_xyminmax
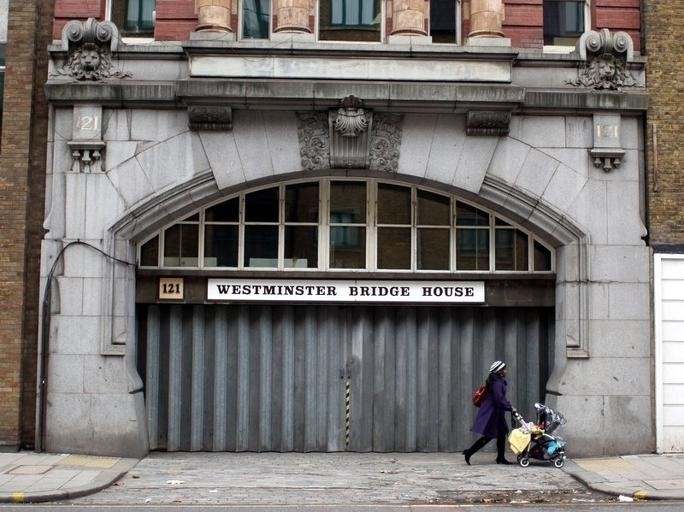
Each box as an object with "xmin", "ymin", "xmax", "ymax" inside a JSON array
[{"xmin": 461, "ymin": 361, "xmax": 517, "ymax": 464}]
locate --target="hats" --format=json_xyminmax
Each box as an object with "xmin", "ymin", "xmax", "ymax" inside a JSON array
[{"xmin": 490, "ymin": 361, "xmax": 506, "ymax": 373}]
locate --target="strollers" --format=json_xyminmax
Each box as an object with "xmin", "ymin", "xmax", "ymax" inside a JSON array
[{"xmin": 509, "ymin": 401, "xmax": 566, "ymax": 467}]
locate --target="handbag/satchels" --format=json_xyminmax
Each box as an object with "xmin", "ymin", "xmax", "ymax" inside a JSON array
[{"xmin": 471, "ymin": 382, "xmax": 492, "ymax": 407}]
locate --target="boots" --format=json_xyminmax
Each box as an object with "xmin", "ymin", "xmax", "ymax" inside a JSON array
[
  {"xmin": 494, "ymin": 435, "xmax": 514, "ymax": 465},
  {"xmin": 461, "ymin": 436, "xmax": 492, "ymax": 466}
]
